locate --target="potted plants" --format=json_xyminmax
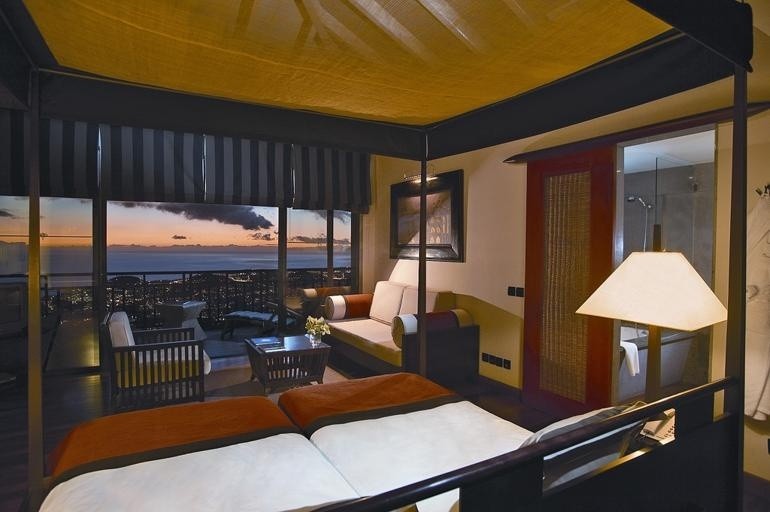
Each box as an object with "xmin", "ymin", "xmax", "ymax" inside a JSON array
[{"xmin": 305, "ymin": 314, "xmax": 331, "ymax": 348}]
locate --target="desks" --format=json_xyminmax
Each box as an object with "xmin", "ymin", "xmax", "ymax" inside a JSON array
[{"xmin": 154, "ymin": 298, "xmax": 209, "ymax": 343}]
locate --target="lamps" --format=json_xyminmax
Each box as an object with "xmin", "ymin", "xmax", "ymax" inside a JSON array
[{"xmin": 574, "ymin": 249, "xmax": 729, "ymax": 406}]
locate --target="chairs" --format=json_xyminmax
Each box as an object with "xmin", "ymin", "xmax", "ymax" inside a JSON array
[
  {"xmin": 102, "ymin": 311, "xmax": 211, "ymax": 411},
  {"xmin": 218, "ymin": 308, "xmax": 297, "ymax": 340}
]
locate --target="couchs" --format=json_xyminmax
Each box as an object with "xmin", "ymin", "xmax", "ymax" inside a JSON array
[
  {"xmin": 275, "ymin": 284, "xmax": 352, "ymax": 313},
  {"xmin": 316, "ymin": 280, "xmax": 480, "ymax": 385}
]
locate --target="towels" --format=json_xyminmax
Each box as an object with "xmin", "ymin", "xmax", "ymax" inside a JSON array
[{"xmin": 619, "ymin": 338, "xmax": 644, "ymax": 377}]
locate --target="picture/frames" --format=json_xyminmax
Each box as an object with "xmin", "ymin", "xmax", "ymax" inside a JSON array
[{"xmin": 387, "ymin": 168, "xmax": 467, "ymax": 263}]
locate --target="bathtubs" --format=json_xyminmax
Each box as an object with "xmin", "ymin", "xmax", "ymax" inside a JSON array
[{"xmin": 620, "ymin": 325, "xmax": 701, "ymax": 402}]
